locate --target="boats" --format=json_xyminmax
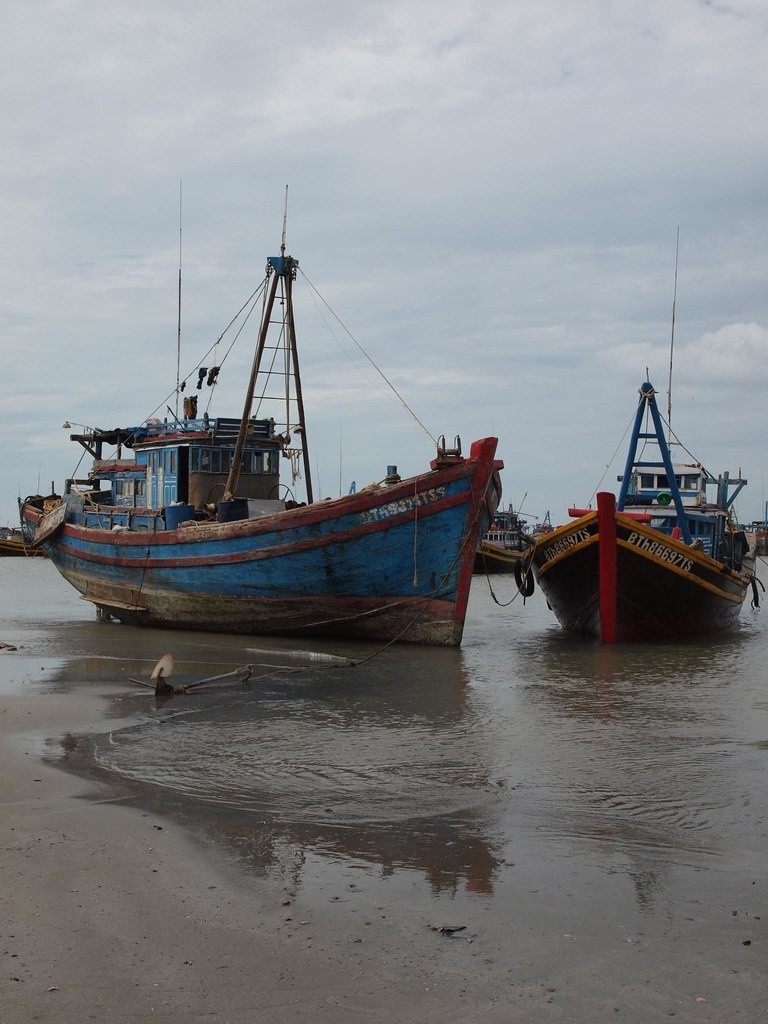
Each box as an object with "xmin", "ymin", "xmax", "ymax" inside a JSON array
[
  {"xmin": 17, "ymin": 180, "xmax": 505, "ymax": 649},
  {"xmin": 0, "ymin": 526, "xmax": 45, "ymax": 556},
  {"xmin": 751, "ymin": 500, "xmax": 768, "ymax": 556},
  {"xmin": 472, "ymin": 491, "xmax": 554, "ymax": 575},
  {"xmin": 523, "ymin": 227, "xmax": 758, "ymax": 648}
]
